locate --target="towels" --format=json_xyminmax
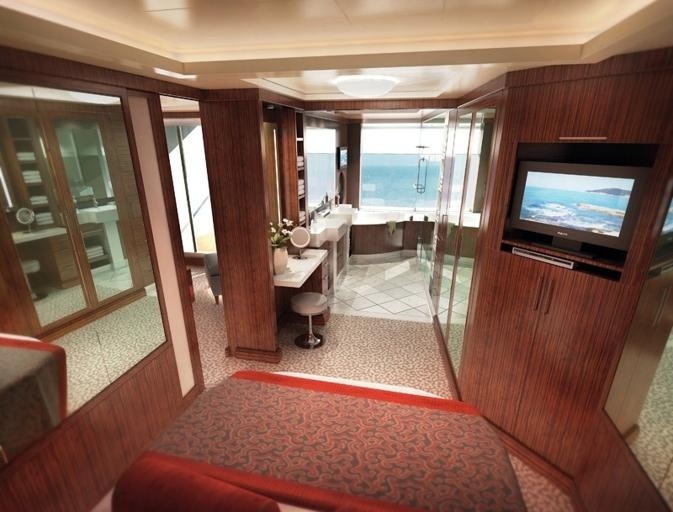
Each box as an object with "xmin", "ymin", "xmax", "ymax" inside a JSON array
[{"xmin": 387, "ymin": 220, "xmax": 396, "ymax": 234}]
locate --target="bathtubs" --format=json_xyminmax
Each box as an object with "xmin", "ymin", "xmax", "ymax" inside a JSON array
[{"xmin": 353, "ymin": 210, "xmax": 410, "ymax": 224}]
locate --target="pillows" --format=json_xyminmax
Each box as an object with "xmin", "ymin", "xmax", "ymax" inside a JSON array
[{"xmin": 111, "ymin": 458, "xmax": 279, "ymax": 512}]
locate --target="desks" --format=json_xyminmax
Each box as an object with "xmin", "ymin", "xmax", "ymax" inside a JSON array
[
  {"xmin": 273, "ymin": 247, "xmax": 328, "ymax": 288},
  {"xmin": 0, "ymin": 332, "xmax": 67, "ymax": 470},
  {"xmin": 12, "ymin": 227, "xmax": 66, "ymax": 246}
]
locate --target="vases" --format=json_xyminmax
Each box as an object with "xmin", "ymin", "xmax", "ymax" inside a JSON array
[{"xmin": 274, "ymin": 247, "xmax": 288, "ymax": 274}]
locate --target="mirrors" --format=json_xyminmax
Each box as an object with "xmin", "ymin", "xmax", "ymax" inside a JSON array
[
  {"xmin": 304, "ymin": 127, "xmax": 336, "ymax": 225},
  {"xmin": 16, "ymin": 208, "xmax": 35, "ymax": 233},
  {"xmin": 290, "ymin": 226, "xmax": 310, "ymax": 260},
  {"xmin": 600, "ymin": 198, "xmax": 673, "ymax": 511}
]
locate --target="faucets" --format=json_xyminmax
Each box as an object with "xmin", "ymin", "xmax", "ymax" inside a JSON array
[{"xmin": 329, "ymin": 198, "xmax": 334, "ymax": 209}]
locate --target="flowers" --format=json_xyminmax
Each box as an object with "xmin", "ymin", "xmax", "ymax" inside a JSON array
[{"xmin": 270, "ymin": 219, "xmax": 293, "ymax": 247}]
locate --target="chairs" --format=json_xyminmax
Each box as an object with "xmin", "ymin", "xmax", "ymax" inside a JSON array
[{"xmin": 204, "ymin": 253, "xmax": 222, "ymax": 305}]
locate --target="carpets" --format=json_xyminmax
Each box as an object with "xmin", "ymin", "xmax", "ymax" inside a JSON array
[
  {"xmin": 0, "ymin": 286, "xmax": 573, "ymax": 512},
  {"xmin": 37, "ymin": 287, "xmax": 167, "ymax": 419}
]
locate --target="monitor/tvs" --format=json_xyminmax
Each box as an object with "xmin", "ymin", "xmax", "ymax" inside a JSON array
[
  {"xmin": 508, "ymin": 160, "xmax": 652, "ymax": 260},
  {"xmin": 337, "ymin": 147, "xmax": 349, "ymax": 170}
]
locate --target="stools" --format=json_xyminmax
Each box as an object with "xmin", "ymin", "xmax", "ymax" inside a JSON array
[
  {"xmin": 22, "ymin": 260, "xmax": 40, "ymax": 300},
  {"xmin": 292, "ymin": 293, "xmax": 328, "ymax": 348}
]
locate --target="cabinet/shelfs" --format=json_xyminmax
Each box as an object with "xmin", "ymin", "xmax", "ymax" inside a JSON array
[
  {"xmin": 0, "ymin": 117, "xmax": 60, "ymax": 230},
  {"xmin": 302, "ymin": 244, "xmax": 330, "ymax": 325},
  {"xmin": 41, "ymin": 223, "xmax": 110, "ymax": 288},
  {"xmin": 603, "ymin": 201, "xmax": 673, "ymax": 442},
  {"xmin": 279, "ymin": 107, "xmax": 306, "ymax": 224},
  {"xmin": 459, "ymin": 46, "xmax": 672, "ymax": 487}
]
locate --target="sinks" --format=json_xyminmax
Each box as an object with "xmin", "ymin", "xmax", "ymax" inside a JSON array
[{"xmin": 331, "ymin": 207, "xmax": 358, "ymax": 225}]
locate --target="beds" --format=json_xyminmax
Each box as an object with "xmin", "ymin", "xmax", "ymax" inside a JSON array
[{"xmin": 87, "ymin": 370, "xmax": 524, "ymax": 512}]
